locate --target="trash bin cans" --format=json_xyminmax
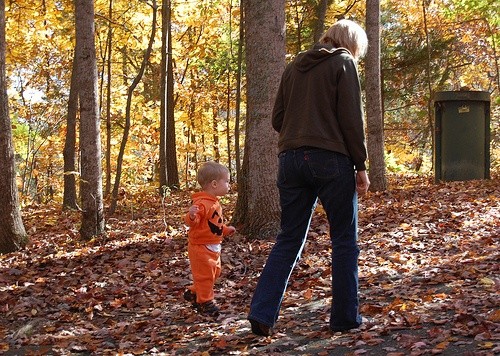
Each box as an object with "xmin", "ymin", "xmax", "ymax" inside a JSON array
[{"xmin": 432, "ymin": 91, "xmax": 490, "ymax": 184}]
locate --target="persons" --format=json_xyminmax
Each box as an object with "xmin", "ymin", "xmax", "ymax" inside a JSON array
[
  {"xmin": 183, "ymin": 162, "xmax": 236, "ymax": 313},
  {"xmin": 247, "ymin": 18, "xmax": 371, "ymax": 337}
]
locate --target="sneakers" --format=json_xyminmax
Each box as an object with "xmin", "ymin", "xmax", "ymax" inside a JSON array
[
  {"xmin": 193, "ymin": 300, "xmax": 219, "ymax": 317},
  {"xmin": 184, "ymin": 290, "xmax": 196, "ymax": 304}
]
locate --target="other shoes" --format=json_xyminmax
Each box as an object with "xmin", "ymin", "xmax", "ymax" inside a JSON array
[
  {"xmin": 249, "ymin": 317, "xmax": 279, "ymax": 337},
  {"xmin": 352, "ymin": 321, "xmax": 373, "ymax": 332}
]
